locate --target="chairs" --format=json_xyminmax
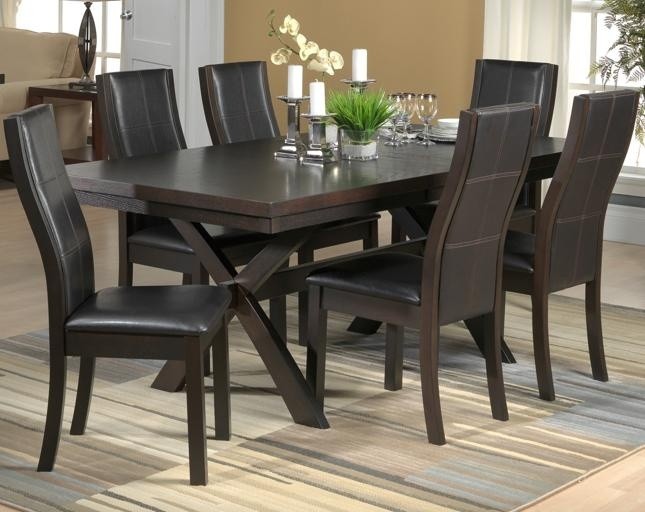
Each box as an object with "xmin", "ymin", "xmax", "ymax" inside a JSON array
[
  {"xmin": 500, "ymin": 88, "xmax": 641, "ymax": 403},
  {"xmin": 94, "ymin": 67, "xmax": 293, "ymax": 378},
  {"xmin": 3, "ymin": 103, "xmax": 231, "ymax": 486},
  {"xmin": 391, "ymin": 58, "xmax": 559, "ymax": 248},
  {"xmin": 296, "ymin": 101, "xmax": 542, "ymax": 447},
  {"xmin": 197, "ymin": 59, "xmax": 382, "ymax": 346}
]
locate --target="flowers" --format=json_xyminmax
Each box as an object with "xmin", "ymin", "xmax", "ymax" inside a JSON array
[{"xmin": 264, "ymin": 8, "xmax": 344, "ymax": 80}]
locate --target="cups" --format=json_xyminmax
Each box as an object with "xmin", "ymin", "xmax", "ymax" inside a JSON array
[
  {"xmin": 415, "ymin": 93, "xmax": 439, "ymax": 146},
  {"xmin": 382, "ymin": 94, "xmax": 406, "ymax": 147},
  {"xmin": 396, "ymin": 92, "xmax": 416, "ymax": 143}
]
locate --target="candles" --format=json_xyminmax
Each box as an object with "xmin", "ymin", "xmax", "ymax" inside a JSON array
[
  {"xmin": 309, "ymin": 79, "xmax": 326, "ymax": 115},
  {"xmin": 287, "ymin": 64, "xmax": 304, "ymax": 99},
  {"xmin": 351, "ymin": 49, "xmax": 368, "ymax": 83}
]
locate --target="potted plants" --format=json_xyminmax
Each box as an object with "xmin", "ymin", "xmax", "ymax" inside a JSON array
[{"xmin": 318, "ymin": 79, "xmax": 400, "ymax": 161}]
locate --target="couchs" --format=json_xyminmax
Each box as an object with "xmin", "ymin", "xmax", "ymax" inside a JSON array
[{"xmin": 0, "ymin": 27, "xmax": 97, "ymax": 184}]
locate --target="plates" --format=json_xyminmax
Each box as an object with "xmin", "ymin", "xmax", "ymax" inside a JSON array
[{"xmin": 418, "ymin": 127, "xmax": 458, "ymax": 143}]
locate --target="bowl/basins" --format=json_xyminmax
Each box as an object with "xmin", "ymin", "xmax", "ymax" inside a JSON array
[{"xmin": 437, "ymin": 118, "xmax": 460, "ymax": 129}]
